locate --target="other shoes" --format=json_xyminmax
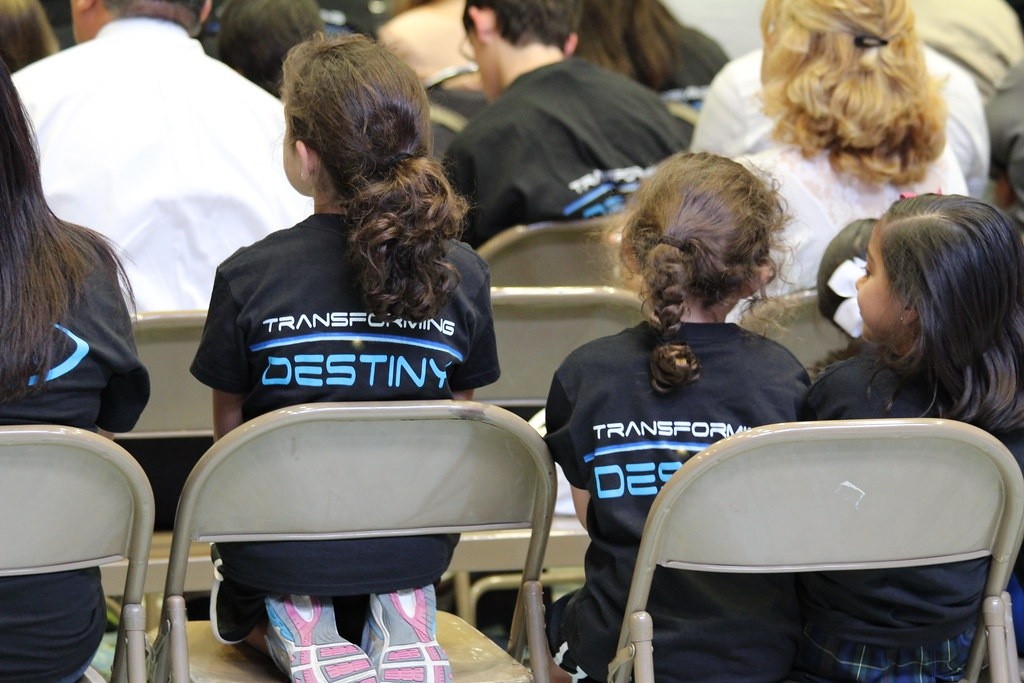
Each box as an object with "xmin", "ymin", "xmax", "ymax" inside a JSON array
[
  {"xmin": 360, "ymin": 581, "xmax": 454, "ymax": 683},
  {"xmin": 263, "ymin": 582, "xmax": 379, "ymax": 683}
]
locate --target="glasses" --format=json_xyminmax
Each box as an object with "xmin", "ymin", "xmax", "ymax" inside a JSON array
[{"xmin": 457, "ymin": 33, "xmax": 478, "ymax": 62}]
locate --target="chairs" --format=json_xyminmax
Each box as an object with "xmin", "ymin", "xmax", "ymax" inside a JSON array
[{"xmin": 1, "ymin": 106, "xmax": 1023, "ymax": 683}]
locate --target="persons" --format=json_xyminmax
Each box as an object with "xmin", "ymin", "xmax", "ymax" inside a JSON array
[
  {"xmin": 0, "ymin": 0, "xmax": 1024, "ymax": 683},
  {"xmin": 186, "ymin": 36, "xmax": 504, "ymax": 683}
]
